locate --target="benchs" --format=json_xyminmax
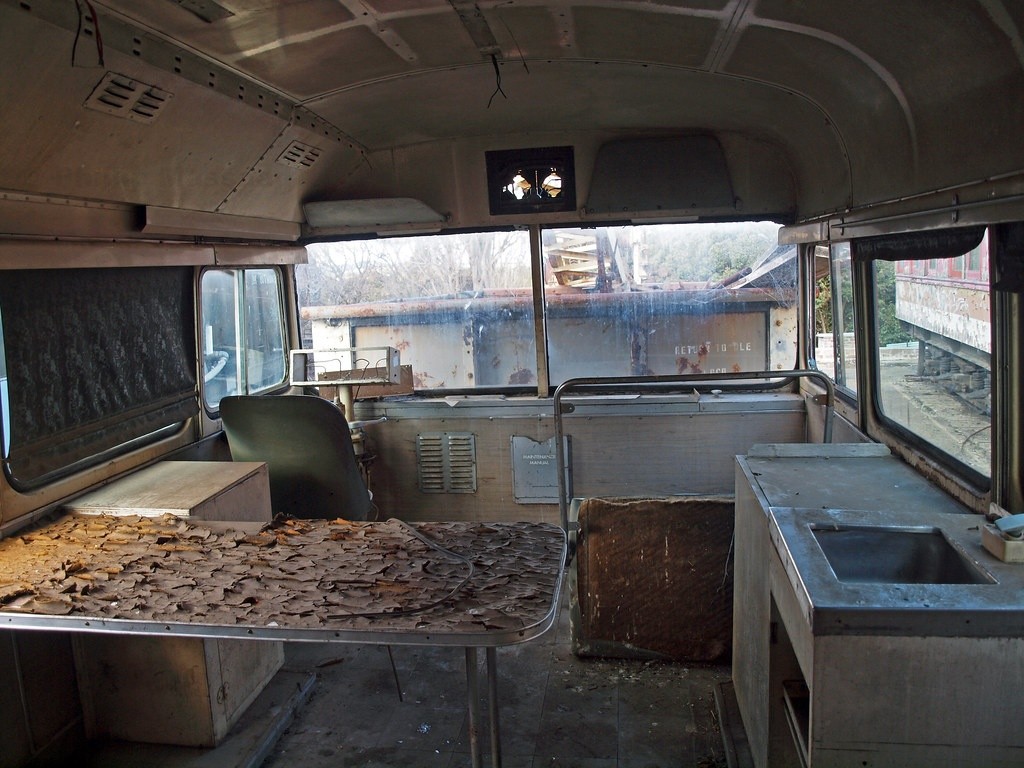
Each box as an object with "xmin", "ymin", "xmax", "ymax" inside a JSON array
[{"xmin": 567, "ymin": 493, "xmax": 736, "ymax": 666}]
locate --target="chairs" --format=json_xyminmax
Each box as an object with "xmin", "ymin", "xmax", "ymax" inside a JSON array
[{"xmin": 219, "ymin": 397, "xmax": 375, "ymax": 523}]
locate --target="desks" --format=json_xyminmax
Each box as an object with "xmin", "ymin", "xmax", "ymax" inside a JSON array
[{"xmin": 0, "ymin": 518, "xmax": 568, "ymax": 768}]
